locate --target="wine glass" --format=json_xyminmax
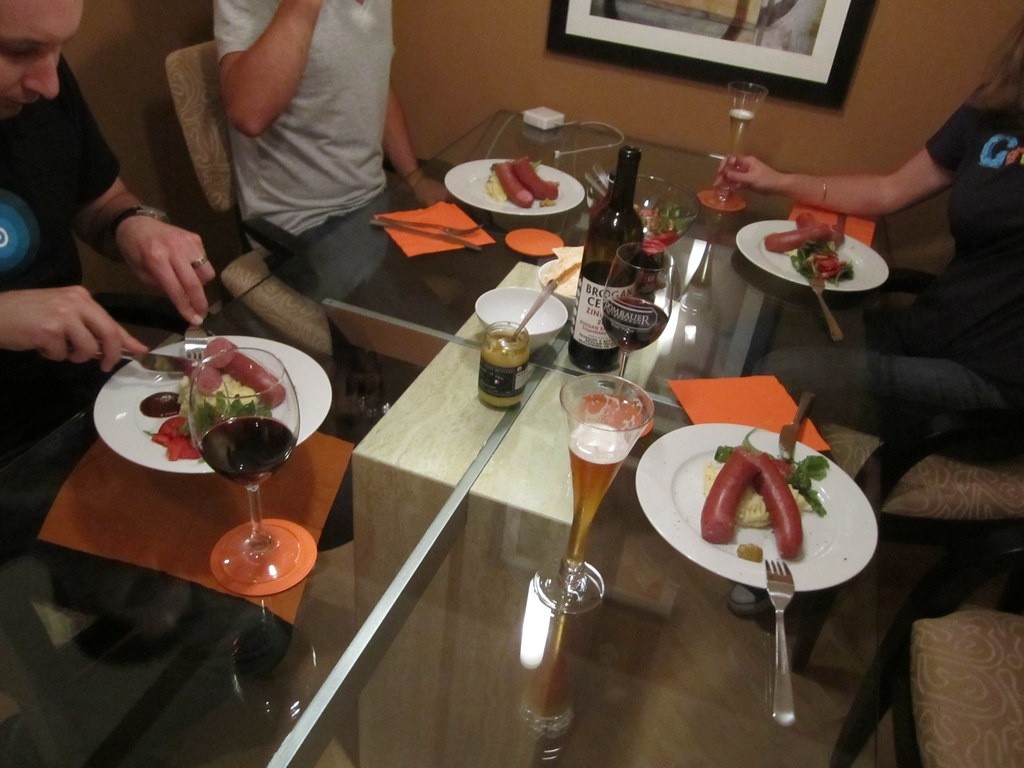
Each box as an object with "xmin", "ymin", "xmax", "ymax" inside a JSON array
[
  {"xmin": 231, "ymin": 597, "xmax": 318, "ymax": 723},
  {"xmin": 186, "ymin": 345, "xmax": 317, "ymax": 596},
  {"xmin": 704, "ymin": 79, "xmax": 767, "ymax": 211},
  {"xmin": 600, "ymin": 243, "xmax": 672, "ymax": 379}
]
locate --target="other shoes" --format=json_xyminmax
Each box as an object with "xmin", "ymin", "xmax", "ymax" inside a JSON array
[
  {"xmin": 47, "ymin": 556, "xmax": 194, "ymax": 635},
  {"xmin": 728, "ymin": 583, "xmax": 774, "ymax": 616},
  {"xmin": 338, "ymin": 366, "xmax": 386, "ymax": 416}
]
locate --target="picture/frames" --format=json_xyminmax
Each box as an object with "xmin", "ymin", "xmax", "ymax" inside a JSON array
[{"xmin": 548, "ymin": 0, "xmax": 875, "ymax": 109}]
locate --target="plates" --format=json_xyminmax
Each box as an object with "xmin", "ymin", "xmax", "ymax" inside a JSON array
[
  {"xmin": 736, "ymin": 220, "xmax": 889, "ymax": 290},
  {"xmin": 635, "ymin": 422, "xmax": 879, "ymax": 591},
  {"xmin": 92, "ymin": 334, "xmax": 332, "ymax": 473},
  {"xmin": 444, "ymin": 158, "xmax": 585, "ymax": 216}
]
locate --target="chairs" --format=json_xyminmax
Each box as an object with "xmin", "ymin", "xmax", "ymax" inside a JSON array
[{"xmin": 86, "ymin": 40, "xmax": 1024, "ymax": 768}]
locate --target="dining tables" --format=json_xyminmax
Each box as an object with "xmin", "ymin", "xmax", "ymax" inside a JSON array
[{"xmin": 0, "ymin": 110, "xmax": 940, "ymax": 768}]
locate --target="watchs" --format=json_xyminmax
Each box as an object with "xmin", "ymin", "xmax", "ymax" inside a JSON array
[{"xmin": 110, "ymin": 203, "xmax": 169, "ymax": 236}]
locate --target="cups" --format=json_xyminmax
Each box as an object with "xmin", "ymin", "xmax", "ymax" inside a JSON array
[
  {"xmin": 532, "ymin": 372, "xmax": 656, "ymax": 616},
  {"xmin": 503, "ymin": 602, "xmax": 605, "ymax": 766}
]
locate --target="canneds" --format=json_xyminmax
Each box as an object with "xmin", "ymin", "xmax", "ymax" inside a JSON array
[{"xmin": 478, "ymin": 320, "xmax": 530, "ymax": 408}]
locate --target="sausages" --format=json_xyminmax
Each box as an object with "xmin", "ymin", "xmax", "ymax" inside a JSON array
[
  {"xmin": 795, "ymin": 211, "xmax": 819, "ymax": 227},
  {"xmin": 700, "ymin": 446, "xmax": 762, "ymax": 543},
  {"xmin": 751, "ymin": 452, "xmax": 803, "ymax": 559},
  {"xmin": 765, "ymin": 222, "xmax": 833, "ymax": 252},
  {"xmin": 494, "ymin": 158, "xmax": 559, "ymax": 207},
  {"xmin": 190, "ymin": 339, "xmax": 285, "ymax": 407}
]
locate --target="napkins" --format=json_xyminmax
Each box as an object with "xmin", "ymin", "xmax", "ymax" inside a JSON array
[
  {"xmin": 662, "ymin": 376, "xmax": 831, "ymax": 456},
  {"xmin": 33, "ymin": 419, "xmax": 353, "ymax": 627},
  {"xmin": 785, "ymin": 203, "xmax": 875, "ymax": 249},
  {"xmin": 371, "ymin": 202, "xmax": 495, "ymax": 259}
]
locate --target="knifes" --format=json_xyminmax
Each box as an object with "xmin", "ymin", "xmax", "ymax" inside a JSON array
[
  {"xmin": 779, "ymin": 391, "xmax": 817, "ymax": 460},
  {"xmin": 67, "ymin": 345, "xmax": 197, "ymax": 373},
  {"xmin": 369, "ymin": 218, "xmax": 481, "ymax": 251}
]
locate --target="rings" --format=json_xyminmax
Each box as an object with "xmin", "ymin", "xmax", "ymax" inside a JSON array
[{"xmin": 191, "ymin": 255, "xmax": 207, "ymax": 269}]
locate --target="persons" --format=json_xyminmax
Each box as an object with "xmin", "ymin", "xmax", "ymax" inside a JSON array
[
  {"xmin": 0, "ymin": 0, "xmax": 352, "ymax": 768},
  {"xmin": 713, "ymin": 14, "xmax": 1024, "ymax": 615},
  {"xmin": 212, "ymin": 1, "xmax": 449, "ymax": 406}
]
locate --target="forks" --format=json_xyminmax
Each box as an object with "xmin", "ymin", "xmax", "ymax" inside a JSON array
[
  {"xmin": 806, "ymin": 276, "xmax": 845, "ymax": 342},
  {"xmin": 183, "ymin": 320, "xmax": 208, "ymax": 362},
  {"xmin": 764, "ymin": 559, "xmax": 798, "ymax": 728},
  {"xmin": 379, "ymin": 216, "xmax": 484, "ymax": 235}
]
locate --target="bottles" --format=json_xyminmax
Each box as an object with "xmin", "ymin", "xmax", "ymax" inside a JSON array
[
  {"xmin": 567, "ymin": 147, "xmax": 645, "ymax": 374},
  {"xmin": 478, "ymin": 321, "xmax": 530, "ymax": 408}
]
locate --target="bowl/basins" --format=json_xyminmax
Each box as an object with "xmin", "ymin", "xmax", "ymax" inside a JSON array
[
  {"xmin": 538, "ymin": 256, "xmax": 582, "ymax": 321},
  {"xmin": 585, "ymin": 168, "xmax": 701, "ymax": 249},
  {"xmin": 475, "ymin": 286, "xmax": 569, "ymax": 356}
]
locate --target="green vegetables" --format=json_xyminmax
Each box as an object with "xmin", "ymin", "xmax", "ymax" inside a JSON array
[
  {"xmin": 714, "ymin": 426, "xmax": 829, "ymax": 517},
  {"xmin": 792, "ymin": 238, "xmax": 854, "ymax": 286},
  {"xmin": 182, "ymin": 380, "xmax": 271, "ymax": 463}
]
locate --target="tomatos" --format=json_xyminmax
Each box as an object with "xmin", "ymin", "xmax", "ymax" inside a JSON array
[{"xmin": 153, "ymin": 415, "xmax": 201, "ymax": 460}]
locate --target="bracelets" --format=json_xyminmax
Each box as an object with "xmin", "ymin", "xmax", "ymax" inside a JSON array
[{"xmin": 820, "ymin": 176, "xmax": 827, "ymax": 201}]
